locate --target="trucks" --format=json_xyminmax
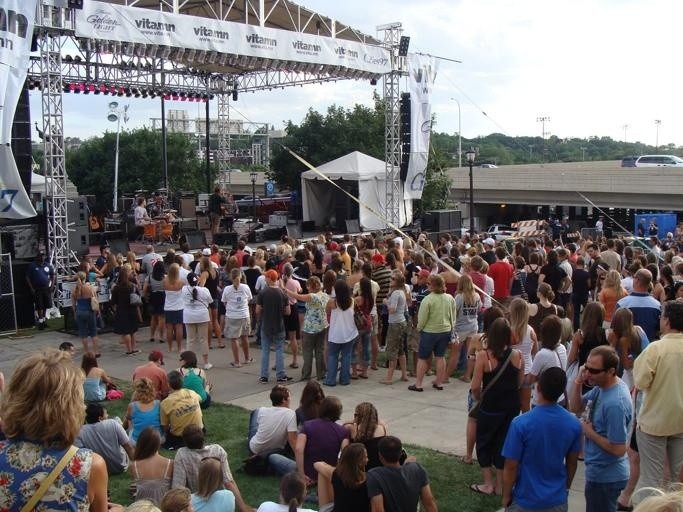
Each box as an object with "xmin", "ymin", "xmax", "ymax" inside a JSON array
[{"xmin": 486, "ymin": 217, "xmax": 556, "ymax": 250}]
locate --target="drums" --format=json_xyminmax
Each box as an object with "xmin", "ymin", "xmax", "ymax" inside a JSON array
[
  {"xmin": 162, "ymin": 224, "xmax": 174, "ymax": 236},
  {"xmin": 144, "ymin": 223, "xmax": 155, "ymax": 237}
]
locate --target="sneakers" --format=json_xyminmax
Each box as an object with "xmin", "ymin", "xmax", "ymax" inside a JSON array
[
  {"xmin": 277, "ymin": 376, "xmax": 294, "ymax": 383},
  {"xmin": 132, "ymin": 350, "xmax": 142, "ymax": 356},
  {"xmin": 290, "ymin": 363, "xmax": 298, "ymax": 368},
  {"xmin": 123, "ymin": 352, "xmax": 132, "ymax": 357},
  {"xmin": 272, "ymin": 367, "xmax": 275, "ymax": 370},
  {"xmin": 38, "ymin": 322, "xmax": 43, "ymax": 329},
  {"xmin": 95, "ymin": 354, "xmax": 100, "ymax": 358},
  {"xmin": 204, "ymin": 363, "xmax": 212, "ymax": 369},
  {"xmin": 258, "ymin": 376, "xmax": 268, "ymax": 384},
  {"xmin": 460, "ymin": 375, "xmax": 470, "ymax": 383},
  {"xmin": 43, "ymin": 321, "xmax": 47, "ymax": 326},
  {"xmin": 400, "ymin": 377, "xmax": 408, "ymax": 381}
]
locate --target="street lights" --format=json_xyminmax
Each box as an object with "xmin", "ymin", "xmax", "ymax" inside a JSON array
[{"xmin": 247, "ymin": 170, "xmax": 261, "ymax": 223}]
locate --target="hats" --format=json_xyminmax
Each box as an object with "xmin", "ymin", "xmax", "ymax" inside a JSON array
[
  {"xmin": 149, "ymin": 351, "xmax": 164, "ymax": 365},
  {"xmin": 482, "ymin": 237, "xmax": 495, "ymax": 246},
  {"xmin": 36, "ymin": 250, "xmax": 44, "ymax": 257},
  {"xmin": 202, "ymin": 248, "xmax": 212, "ymax": 255},
  {"xmin": 371, "ymin": 254, "xmax": 384, "ymax": 262},
  {"xmin": 262, "ymin": 270, "xmax": 278, "ymax": 280},
  {"xmin": 415, "ymin": 269, "xmax": 429, "ymax": 278}
]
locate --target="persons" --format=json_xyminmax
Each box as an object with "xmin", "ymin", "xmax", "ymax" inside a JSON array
[
  {"xmin": 35, "ymin": 124, "xmax": 65, "ymax": 181},
  {"xmin": 0, "ymin": 183, "xmax": 683, "ymax": 512}
]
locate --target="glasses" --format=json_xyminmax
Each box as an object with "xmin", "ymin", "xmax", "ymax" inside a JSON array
[
  {"xmin": 674, "ymin": 280, "xmax": 682, "ymax": 285},
  {"xmin": 585, "ymin": 365, "xmax": 604, "ymax": 374}
]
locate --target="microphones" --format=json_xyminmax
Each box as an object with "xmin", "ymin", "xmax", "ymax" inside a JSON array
[{"xmin": 161, "ymin": 198, "xmax": 167, "ymax": 204}]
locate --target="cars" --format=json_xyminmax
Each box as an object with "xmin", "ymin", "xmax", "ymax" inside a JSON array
[
  {"xmin": 635, "ymin": 155, "xmax": 682, "ymax": 167},
  {"xmin": 621, "ymin": 155, "xmax": 634, "ymax": 168},
  {"xmin": 477, "ymin": 161, "xmax": 499, "ymax": 170}
]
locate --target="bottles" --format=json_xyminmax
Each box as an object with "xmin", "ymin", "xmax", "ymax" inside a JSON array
[{"xmin": 579, "ymin": 398, "xmax": 594, "ymax": 427}]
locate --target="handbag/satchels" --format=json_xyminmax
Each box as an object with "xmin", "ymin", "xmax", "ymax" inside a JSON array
[
  {"xmin": 106, "ymin": 389, "xmax": 123, "ymax": 401},
  {"xmin": 354, "ymin": 302, "xmax": 367, "ymax": 329},
  {"xmin": 242, "ymin": 448, "xmax": 285, "ymax": 476},
  {"xmin": 468, "ymin": 390, "xmax": 481, "ymax": 416},
  {"xmin": 130, "ymin": 284, "xmax": 143, "ymax": 305},
  {"xmin": 145, "ymin": 275, "xmax": 151, "ymax": 303},
  {"xmin": 91, "ymin": 292, "xmax": 99, "ymax": 311},
  {"xmin": 448, "ymin": 329, "xmax": 459, "ymax": 345}
]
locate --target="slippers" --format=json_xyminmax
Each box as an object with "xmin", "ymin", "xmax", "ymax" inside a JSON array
[
  {"xmin": 433, "ymin": 384, "xmax": 443, "ymax": 390},
  {"xmin": 229, "ymin": 362, "xmax": 240, "ymax": 368},
  {"xmin": 243, "ymin": 358, "xmax": 255, "ymax": 365},
  {"xmin": 460, "ymin": 457, "xmax": 473, "ymax": 465},
  {"xmin": 379, "ymin": 379, "xmax": 392, "ymax": 384},
  {"xmin": 408, "ymin": 384, "xmax": 423, "ymax": 392},
  {"xmin": 470, "ymin": 484, "xmax": 487, "ymax": 494}
]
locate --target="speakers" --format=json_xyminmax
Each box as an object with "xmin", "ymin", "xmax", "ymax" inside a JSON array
[
  {"xmin": 247, "ymin": 229, "xmax": 266, "ymax": 244},
  {"xmin": 448, "ymin": 209, "xmax": 462, "ymax": 232},
  {"xmin": 44, "ymin": 196, "xmax": 88, "ymax": 226},
  {"xmin": 266, "ymin": 226, "xmax": 287, "ymax": 240},
  {"xmin": 58, "ymin": 226, "xmax": 89, "ymax": 257},
  {"xmin": 179, "ymin": 230, "xmax": 208, "ymax": 250},
  {"xmin": 101, "ymin": 236, "xmax": 131, "ymax": 257},
  {"xmin": 10, "ymin": 81, "xmax": 31, "ymax": 199},
  {"xmin": 339, "ymin": 219, "xmax": 360, "ymax": 234},
  {"xmin": 396, "ymin": 92, "xmax": 411, "ymax": 180},
  {"xmin": 423, "ymin": 211, "xmax": 451, "ymax": 232},
  {"xmin": 213, "ymin": 232, "xmax": 238, "ymax": 245},
  {"xmin": 302, "ymin": 220, "xmax": 315, "ymax": 232}
]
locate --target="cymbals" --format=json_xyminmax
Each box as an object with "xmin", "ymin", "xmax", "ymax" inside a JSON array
[
  {"xmin": 154, "ymin": 216, "xmax": 168, "ymax": 219},
  {"xmin": 164, "ymin": 209, "xmax": 178, "ymax": 213}
]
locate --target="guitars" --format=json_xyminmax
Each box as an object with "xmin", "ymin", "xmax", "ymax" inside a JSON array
[
  {"xmin": 221, "ymin": 191, "xmax": 225, "ymax": 216},
  {"xmin": 88, "ymin": 206, "xmax": 99, "ymax": 230}
]
